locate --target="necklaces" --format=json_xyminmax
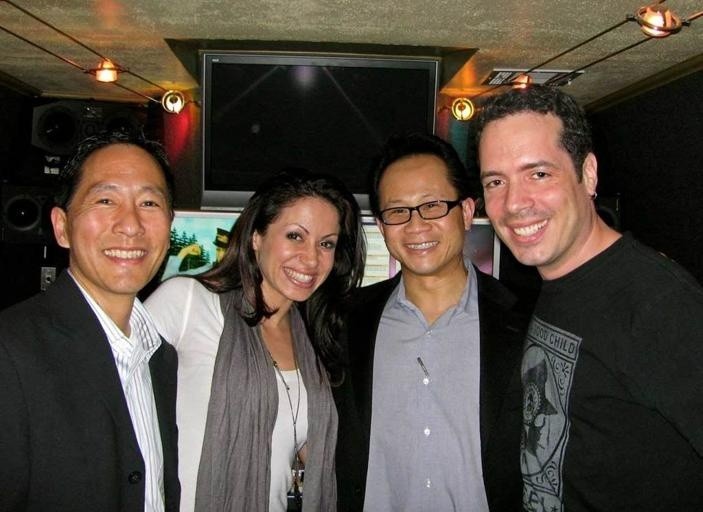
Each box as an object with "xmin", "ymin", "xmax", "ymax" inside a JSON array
[{"xmin": 260, "ymin": 317, "xmax": 304, "ymax": 505}]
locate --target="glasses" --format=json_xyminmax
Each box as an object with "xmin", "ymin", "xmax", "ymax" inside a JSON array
[{"xmin": 378, "ymin": 200, "xmax": 461, "ymax": 227}]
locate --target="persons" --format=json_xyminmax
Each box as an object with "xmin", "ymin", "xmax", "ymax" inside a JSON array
[
  {"xmin": 0, "ymin": 128, "xmax": 183, "ymax": 512},
  {"xmin": 317, "ymin": 133, "xmax": 524, "ymax": 512},
  {"xmin": 141, "ymin": 176, "xmax": 367, "ymax": 512},
  {"xmin": 467, "ymin": 83, "xmax": 701, "ymax": 512}
]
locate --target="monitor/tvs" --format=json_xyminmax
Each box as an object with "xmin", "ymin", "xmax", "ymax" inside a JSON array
[{"xmin": 197, "ymin": 48, "xmax": 442, "ymax": 215}]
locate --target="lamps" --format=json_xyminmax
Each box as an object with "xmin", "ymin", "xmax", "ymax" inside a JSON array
[
  {"xmin": 83, "ymin": 55, "xmax": 125, "ymax": 84},
  {"xmin": 160, "ymin": 89, "xmax": 186, "ymax": 116},
  {"xmin": 626, "ymin": 3, "xmax": 692, "ymax": 39},
  {"xmin": 451, "ymin": 96, "xmax": 477, "ymax": 122}
]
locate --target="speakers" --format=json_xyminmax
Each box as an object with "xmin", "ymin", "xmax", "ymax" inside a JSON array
[{"xmin": 0, "ymin": 96, "xmax": 166, "ymax": 245}]
